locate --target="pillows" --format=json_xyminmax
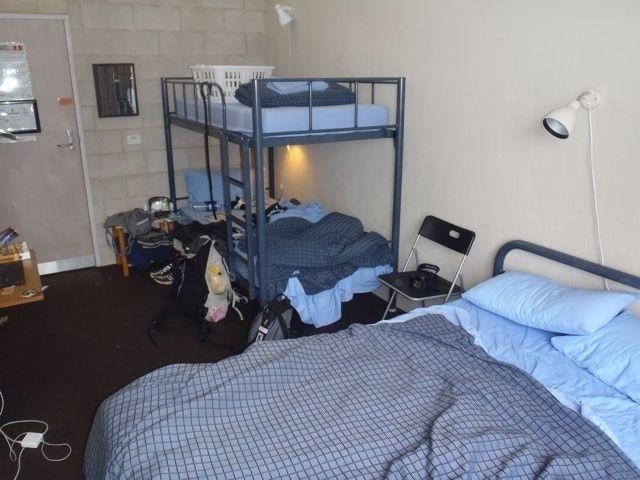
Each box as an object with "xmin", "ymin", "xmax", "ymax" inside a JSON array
[
  {"xmin": 183, "ymin": 166, "xmax": 244, "ymax": 209},
  {"xmin": 462, "ymin": 268, "xmax": 640, "ymax": 336},
  {"xmin": 550, "ymin": 308, "xmax": 640, "ymax": 403}
]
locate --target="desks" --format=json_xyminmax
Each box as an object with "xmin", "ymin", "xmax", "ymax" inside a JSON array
[{"xmin": 0, "ymin": 248, "xmax": 45, "ymax": 309}]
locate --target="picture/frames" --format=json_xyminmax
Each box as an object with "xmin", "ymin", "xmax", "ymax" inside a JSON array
[{"xmin": 0, "ymin": 99, "xmax": 41, "ymax": 134}]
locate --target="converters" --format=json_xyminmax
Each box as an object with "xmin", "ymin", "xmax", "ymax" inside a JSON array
[{"xmin": 21, "ymin": 432, "xmax": 43, "ymax": 448}]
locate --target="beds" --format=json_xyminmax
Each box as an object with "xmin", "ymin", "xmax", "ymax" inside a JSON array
[
  {"xmin": 81, "ymin": 239, "xmax": 640, "ymax": 480},
  {"xmin": 161, "ymin": 76, "xmax": 406, "ymax": 317}
]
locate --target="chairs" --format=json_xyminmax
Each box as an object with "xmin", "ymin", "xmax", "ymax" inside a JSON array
[{"xmin": 378, "ymin": 215, "xmax": 476, "ymax": 320}]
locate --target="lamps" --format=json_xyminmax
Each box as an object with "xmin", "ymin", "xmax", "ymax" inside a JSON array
[
  {"xmin": 541, "ymin": 90, "xmax": 609, "ymax": 291},
  {"xmin": 274, "ymin": 3, "xmax": 293, "ymax": 28}
]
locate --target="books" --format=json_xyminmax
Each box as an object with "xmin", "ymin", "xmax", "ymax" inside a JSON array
[{"xmin": 0, "ymin": 241, "xmax": 31, "ymax": 264}]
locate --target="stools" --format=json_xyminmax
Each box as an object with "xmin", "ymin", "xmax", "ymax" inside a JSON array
[{"xmin": 112, "ymin": 219, "xmax": 169, "ymax": 277}]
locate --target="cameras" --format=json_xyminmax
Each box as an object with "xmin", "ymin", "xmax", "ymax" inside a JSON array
[{"xmin": 408, "ymin": 272, "xmax": 432, "ymax": 291}]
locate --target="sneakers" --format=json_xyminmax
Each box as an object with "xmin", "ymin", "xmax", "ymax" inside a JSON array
[
  {"xmin": 154, "ymin": 274, "xmax": 175, "ymax": 285},
  {"xmin": 150, "ymin": 265, "xmax": 171, "ymax": 278}
]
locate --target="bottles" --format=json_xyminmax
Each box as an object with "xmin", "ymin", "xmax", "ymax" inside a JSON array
[{"xmin": 192, "ymin": 201, "xmax": 224, "ymax": 211}]
